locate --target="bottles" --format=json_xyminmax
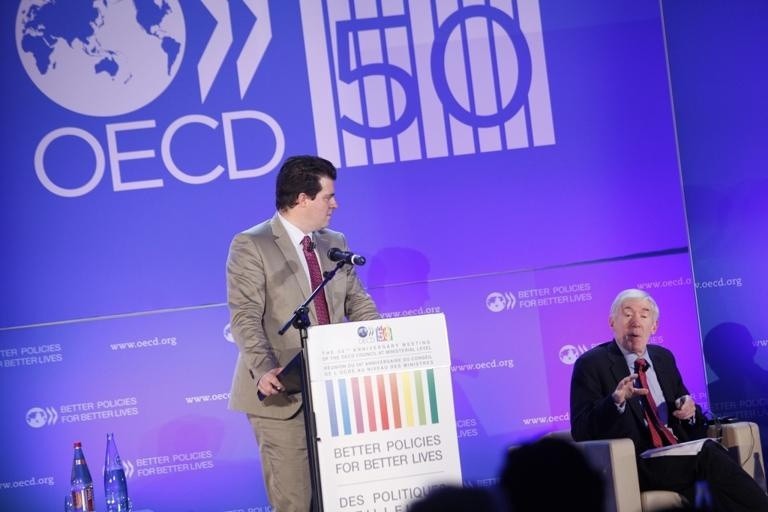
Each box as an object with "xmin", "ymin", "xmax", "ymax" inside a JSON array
[
  {"xmin": 103, "ymin": 432, "xmax": 130, "ymax": 512},
  {"xmin": 69, "ymin": 440, "xmax": 96, "ymax": 512}
]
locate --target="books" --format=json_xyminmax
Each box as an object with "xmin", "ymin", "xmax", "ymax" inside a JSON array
[{"xmin": 638, "ymin": 434, "xmax": 729, "ymax": 460}]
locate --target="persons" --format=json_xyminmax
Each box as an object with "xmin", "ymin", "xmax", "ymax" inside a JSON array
[
  {"xmin": 492, "ymin": 431, "xmax": 609, "ymax": 512},
  {"xmin": 223, "ymin": 153, "xmax": 382, "ymax": 510},
  {"xmin": 568, "ymin": 287, "xmax": 768, "ymax": 509}
]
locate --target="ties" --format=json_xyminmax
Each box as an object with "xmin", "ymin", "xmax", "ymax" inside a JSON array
[
  {"xmin": 301, "ymin": 236, "xmax": 330, "ymax": 324},
  {"xmin": 634, "ymin": 359, "xmax": 677, "ymax": 447}
]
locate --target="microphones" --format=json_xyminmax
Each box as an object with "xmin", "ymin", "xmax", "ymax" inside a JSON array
[
  {"xmin": 644, "ymin": 364, "xmax": 651, "ymax": 372},
  {"xmin": 327, "ymin": 248, "xmax": 366, "ymax": 265}
]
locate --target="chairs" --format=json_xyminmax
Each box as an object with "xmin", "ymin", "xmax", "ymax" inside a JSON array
[{"xmin": 557, "ymin": 422, "xmax": 768, "ymax": 512}]
